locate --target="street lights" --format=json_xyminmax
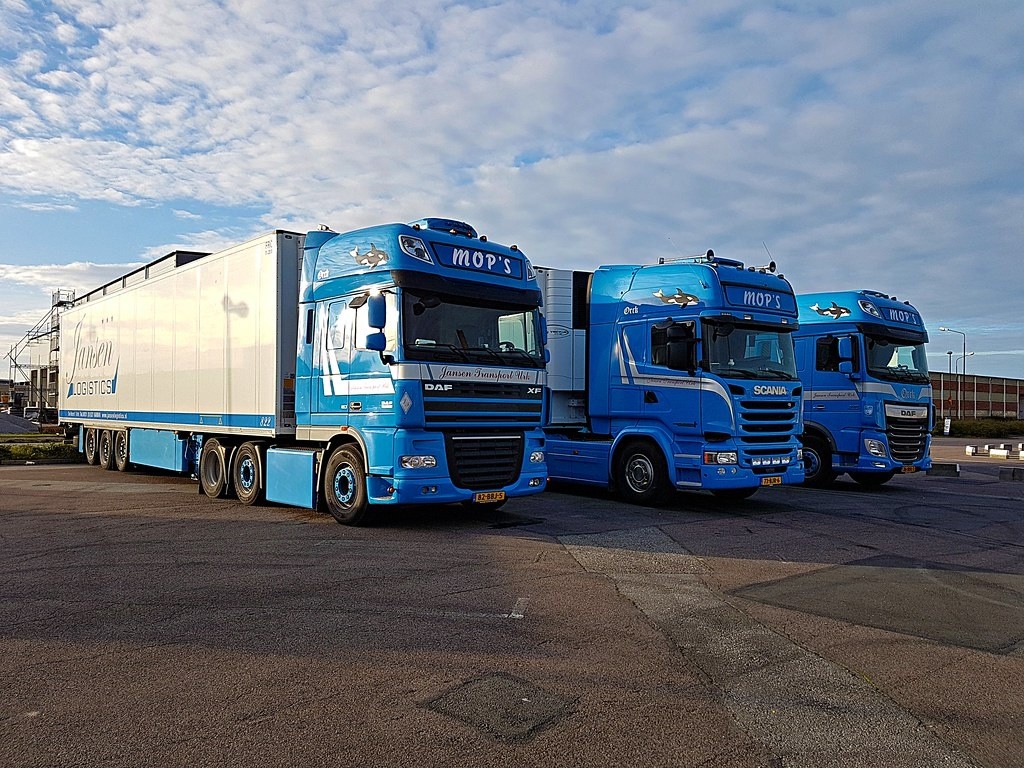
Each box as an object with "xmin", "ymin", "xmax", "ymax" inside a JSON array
[
  {"xmin": 939, "ymin": 326, "xmax": 966, "ymax": 421},
  {"xmin": 954, "ymin": 351, "xmax": 975, "ymax": 420}
]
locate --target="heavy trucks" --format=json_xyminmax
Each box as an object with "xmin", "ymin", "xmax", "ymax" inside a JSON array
[
  {"xmin": 56, "ymin": 220, "xmax": 552, "ymax": 528},
  {"xmin": 744, "ymin": 289, "xmax": 937, "ymax": 489},
  {"xmin": 501, "ymin": 246, "xmax": 805, "ymax": 502}
]
